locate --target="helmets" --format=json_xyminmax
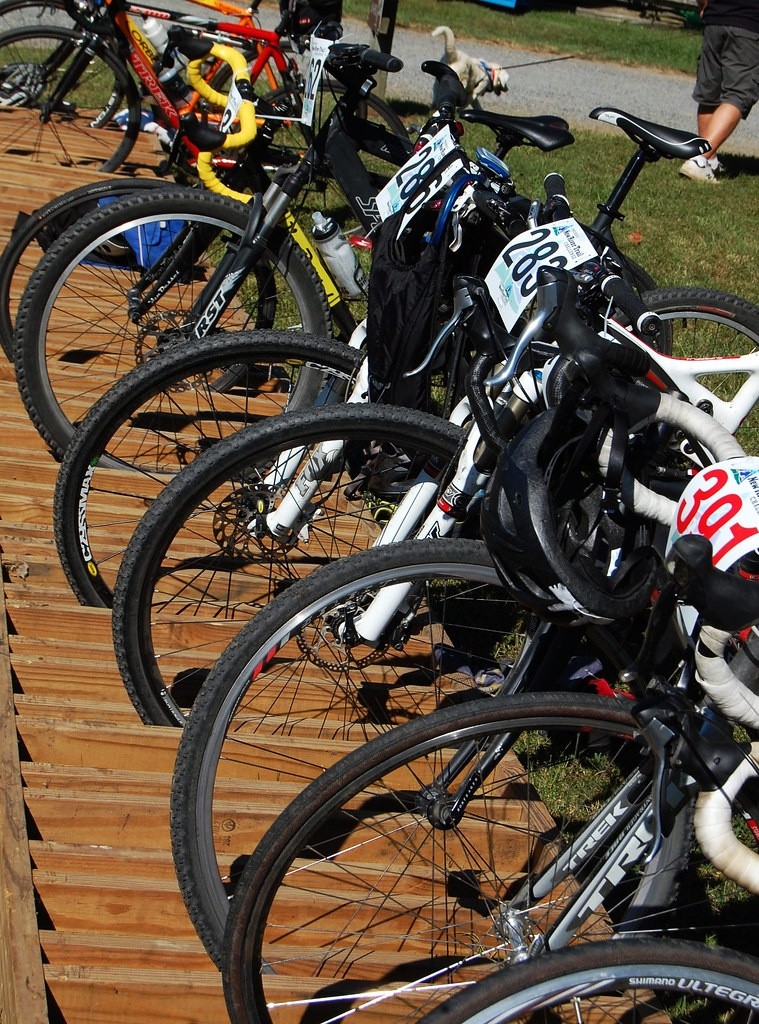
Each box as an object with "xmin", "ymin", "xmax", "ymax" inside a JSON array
[
  {"xmin": 478, "ymin": 376, "xmax": 662, "ymax": 629},
  {"xmin": 663, "ymin": 455, "xmax": 759, "ymax": 638}
]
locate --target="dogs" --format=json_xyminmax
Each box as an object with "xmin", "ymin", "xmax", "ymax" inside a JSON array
[{"xmin": 430, "ymin": 25, "xmax": 509, "ymax": 114}]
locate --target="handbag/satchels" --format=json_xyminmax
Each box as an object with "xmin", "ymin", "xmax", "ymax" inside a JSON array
[{"xmin": 366, "ymin": 148, "xmax": 470, "ymax": 413}]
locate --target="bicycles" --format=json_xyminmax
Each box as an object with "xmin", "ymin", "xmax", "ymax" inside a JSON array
[{"xmin": 1, "ymin": 0, "xmax": 759, "ymax": 1024}]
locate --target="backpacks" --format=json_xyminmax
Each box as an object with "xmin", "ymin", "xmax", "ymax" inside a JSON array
[{"xmin": 12, "ymin": 197, "xmax": 197, "ymax": 284}]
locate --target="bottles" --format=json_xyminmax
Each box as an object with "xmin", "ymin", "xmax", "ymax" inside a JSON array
[
  {"xmin": 143, "ymin": 17, "xmax": 171, "ymax": 54},
  {"xmin": 152, "ymin": 55, "xmax": 193, "ymax": 109},
  {"xmin": 312, "ymin": 211, "xmax": 368, "ymax": 296}
]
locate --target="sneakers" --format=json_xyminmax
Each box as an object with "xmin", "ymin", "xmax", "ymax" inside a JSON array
[{"xmin": 679, "ymin": 160, "xmax": 728, "ymax": 184}]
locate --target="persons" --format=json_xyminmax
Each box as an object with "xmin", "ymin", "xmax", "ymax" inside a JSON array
[{"xmin": 678, "ymin": 0, "xmax": 759, "ymax": 185}]
